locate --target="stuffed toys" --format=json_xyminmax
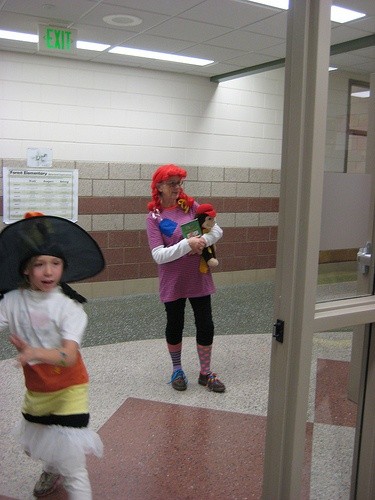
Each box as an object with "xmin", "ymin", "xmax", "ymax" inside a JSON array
[{"xmin": 194, "ymin": 203, "xmax": 219, "ymax": 266}]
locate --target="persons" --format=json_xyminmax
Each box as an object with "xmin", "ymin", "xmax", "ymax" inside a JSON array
[
  {"xmin": 146, "ymin": 164, "xmax": 227, "ymax": 392},
  {"xmin": 0, "ymin": 241, "xmax": 103, "ymax": 500}
]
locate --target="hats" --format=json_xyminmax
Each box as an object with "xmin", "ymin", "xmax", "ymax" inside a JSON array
[{"xmin": 0, "ymin": 212, "xmax": 105, "ymax": 294}]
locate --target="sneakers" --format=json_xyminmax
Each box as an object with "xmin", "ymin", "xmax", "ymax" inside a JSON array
[
  {"xmin": 34, "ymin": 472, "xmax": 60, "ymax": 497},
  {"xmin": 199, "ymin": 371, "xmax": 226, "ymax": 392},
  {"xmin": 168, "ymin": 369, "xmax": 187, "ymax": 391}
]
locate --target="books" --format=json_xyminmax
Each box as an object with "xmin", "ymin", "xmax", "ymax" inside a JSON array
[{"xmin": 181, "ymin": 217, "xmax": 203, "ymax": 239}]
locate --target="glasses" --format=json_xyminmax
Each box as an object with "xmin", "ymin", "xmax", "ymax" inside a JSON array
[{"xmin": 161, "ymin": 180, "xmax": 183, "ymax": 187}]
